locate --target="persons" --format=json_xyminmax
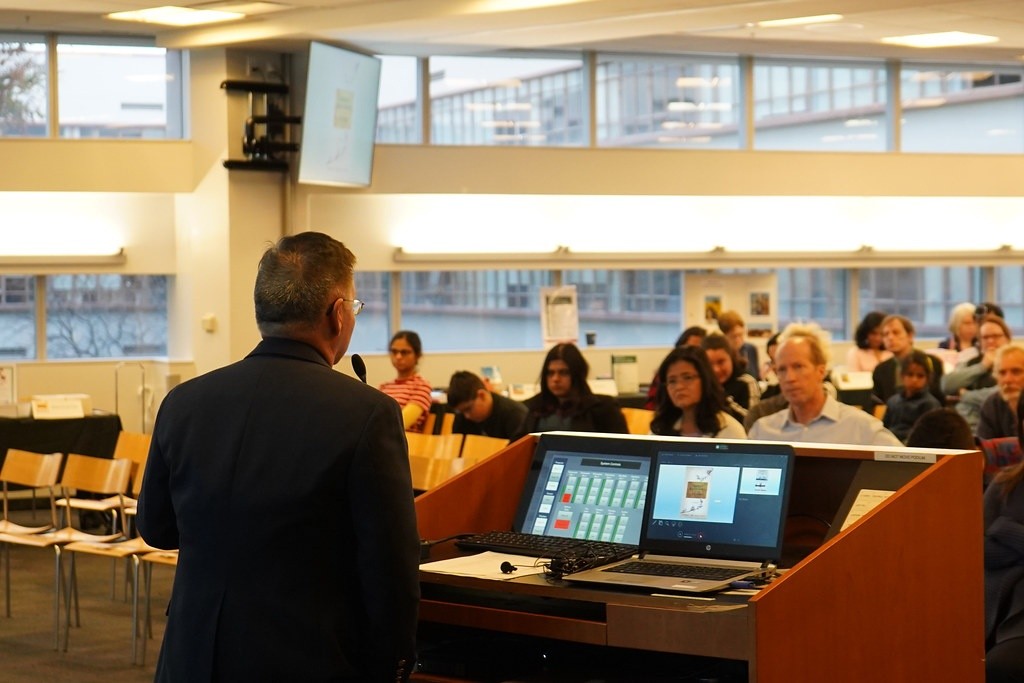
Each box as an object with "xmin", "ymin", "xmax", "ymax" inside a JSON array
[
  {"xmin": 649, "ymin": 345, "xmax": 747, "ymax": 440},
  {"xmin": 379, "ymin": 331, "xmax": 432, "ymax": 433},
  {"xmin": 748, "ymin": 320, "xmax": 910, "ymax": 448},
  {"xmin": 513, "ymin": 341, "xmax": 630, "ymax": 443},
  {"xmin": 648, "ymin": 301, "xmax": 1024, "ymax": 683},
  {"xmin": 135, "ymin": 231, "xmax": 421, "ymax": 683},
  {"xmin": 447, "ymin": 369, "xmax": 528, "ymax": 445}
]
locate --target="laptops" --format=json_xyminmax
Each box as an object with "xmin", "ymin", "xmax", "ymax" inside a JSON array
[{"xmin": 562, "ymin": 441, "xmax": 795, "ymax": 594}]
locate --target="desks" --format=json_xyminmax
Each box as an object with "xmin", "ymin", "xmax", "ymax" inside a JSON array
[{"xmin": 0, "ymin": 412, "xmax": 124, "ymax": 526}]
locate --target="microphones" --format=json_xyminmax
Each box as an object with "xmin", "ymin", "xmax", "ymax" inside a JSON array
[
  {"xmin": 351, "ymin": 354, "xmax": 366, "ymax": 383},
  {"xmin": 500, "ymin": 561, "xmax": 518, "ymax": 573}
]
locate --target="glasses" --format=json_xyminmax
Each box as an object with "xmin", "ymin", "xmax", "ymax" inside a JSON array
[
  {"xmin": 387, "ymin": 349, "xmax": 414, "ymax": 357},
  {"xmin": 345, "ymin": 299, "xmax": 364, "ymax": 314},
  {"xmin": 665, "ymin": 374, "xmax": 701, "ymax": 388}
]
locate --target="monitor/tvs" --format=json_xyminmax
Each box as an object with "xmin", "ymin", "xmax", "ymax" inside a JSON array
[
  {"xmin": 511, "ymin": 434, "xmax": 653, "ymax": 545},
  {"xmin": 297, "ymin": 42, "xmax": 382, "ymax": 189}
]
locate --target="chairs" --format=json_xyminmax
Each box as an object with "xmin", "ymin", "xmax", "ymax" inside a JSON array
[
  {"xmin": 0, "ymin": 431, "xmax": 179, "ymax": 668},
  {"xmin": 405, "ymin": 414, "xmax": 509, "ymax": 492}
]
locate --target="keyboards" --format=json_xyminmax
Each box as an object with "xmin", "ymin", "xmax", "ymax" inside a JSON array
[{"xmin": 454, "ymin": 530, "xmax": 643, "ymax": 565}]
